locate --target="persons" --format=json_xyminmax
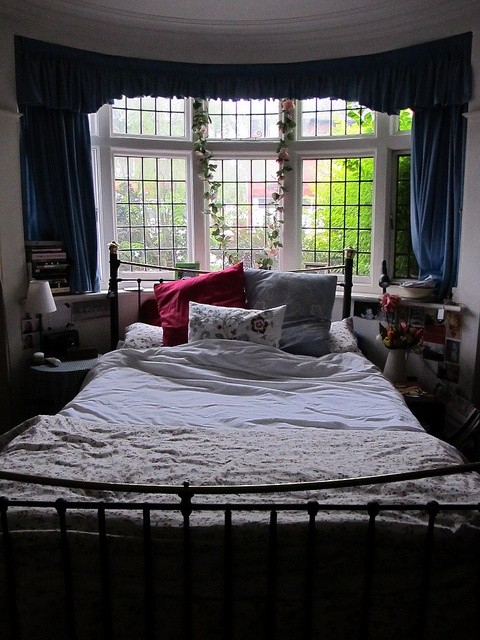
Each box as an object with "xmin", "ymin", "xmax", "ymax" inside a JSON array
[
  {"xmin": 22, "ymin": 335, "xmax": 34, "ymax": 350},
  {"xmin": 450, "ymin": 341, "xmax": 459, "ymax": 362},
  {"xmin": 23, "ymin": 312, "xmax": 33, "ymax": 320},
  {"xmin": 22, "ymin": 321, "xmax": 33, "ymax": 334}
]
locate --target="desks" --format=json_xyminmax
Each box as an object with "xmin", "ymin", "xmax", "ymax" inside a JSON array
[{"xmin": 29, "ymin": 353, "xmax": 104, "ymax": 419}]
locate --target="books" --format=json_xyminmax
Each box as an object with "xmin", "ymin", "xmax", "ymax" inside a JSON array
[
  {"xmin": 35, "ymin": 276, "xmax": 71, "ymax": 295},
  {"xmin": 28, "ymin": 248, "xmax": 69, "ymax": 260}
]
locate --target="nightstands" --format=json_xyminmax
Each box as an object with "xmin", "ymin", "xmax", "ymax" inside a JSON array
[{"xmin": 393, "ymin": 382, "xmax": 449, "ymax": 439}]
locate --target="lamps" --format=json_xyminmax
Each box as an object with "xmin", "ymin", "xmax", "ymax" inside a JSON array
[
  {"xmin": 23, "ymin": 279, "xmax": 58, "ymax": 352},
  {"xmin": 379, "ymin": 259, "xmax": 391, "ymax": 294}
]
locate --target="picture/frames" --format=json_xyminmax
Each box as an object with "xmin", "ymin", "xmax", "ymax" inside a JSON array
[{"xmin": 175, "ymin": 263, "xmax": 200, "ymax": 280}]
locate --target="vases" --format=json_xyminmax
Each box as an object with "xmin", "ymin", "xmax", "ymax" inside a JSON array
[{"xmin": 382, "ymin": 347, "xmax": 408, "ymax": 388}]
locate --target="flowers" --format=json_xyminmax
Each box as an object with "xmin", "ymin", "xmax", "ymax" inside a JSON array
[{"xmin": 375, "ymin": 292, "xmax": 424, "ymax": 349}]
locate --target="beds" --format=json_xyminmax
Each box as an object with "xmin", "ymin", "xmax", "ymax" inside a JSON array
[{"xmin": 1, "ymin": 240, "xmax": 480, "ymax": 640}]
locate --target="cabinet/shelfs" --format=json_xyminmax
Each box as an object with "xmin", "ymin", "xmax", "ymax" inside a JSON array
[
  {"xmin": 42, "ymin": 291, "xmax": 117, "ymax": 348},
  {"xmin": 353, "ymin": 292, "xmax": 461, "ymax": 395}
]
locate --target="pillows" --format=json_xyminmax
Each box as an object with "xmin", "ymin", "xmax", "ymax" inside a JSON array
[
  {"xmin": 328, "ymin": 316, "xmax": 362, "ymax": 355},
  {"xmin": 154, "ymin": 261, "xmax": 250, "ymax": 347},
  {"xmin": 187, "ymin": 300, "xmax": 287, "ymax": 349},
  {"xmin": 243, "ymin": 267, "xmax": 338, "ymax": 357},
  {"xmin": 123, "ymin": 321, "xmax": 164, "ymax": 350}
]
